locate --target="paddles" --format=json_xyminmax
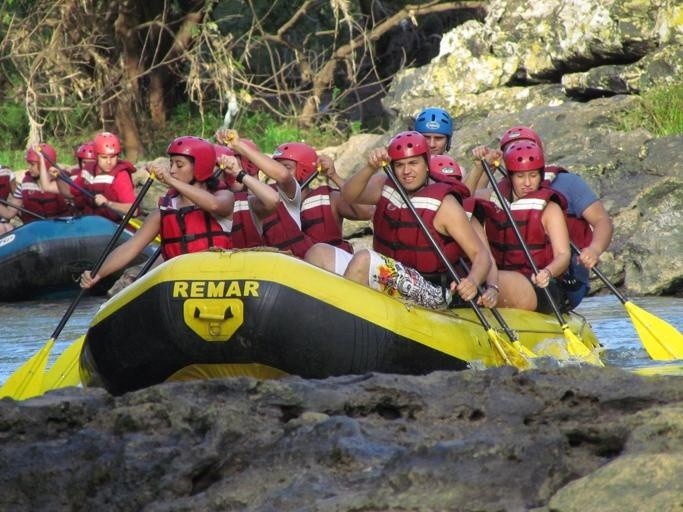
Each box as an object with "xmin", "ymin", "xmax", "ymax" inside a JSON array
[
  {"xmin": 569, "ymin": 237, "xmax": 683, "ymax": 364},
  {"xmin": 478, "ymin": 156, "xmax": 604, "ymax": 368},
  {"xmin": 455, "ymin": 250, "xmax": 539, "ymax": 361},
  {"xmin": 380, "ymin": 159, "xmax": 532, "ymax": 376},
  {"xmin": 0, "ymin": 169, "xmax": 162, "ymax": 402}
]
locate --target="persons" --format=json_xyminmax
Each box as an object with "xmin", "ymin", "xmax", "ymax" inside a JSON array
[
  {"xmin": 74, "ymin": 109, "xmax": 614, "ymax": 325},
  {"xmin": 1, "ymin": 128, "xmax": 141, "ymax": 238}
]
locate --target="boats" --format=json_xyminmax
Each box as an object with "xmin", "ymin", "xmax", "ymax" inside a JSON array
[
  {"xmin": 76, "ymin": 249, "xmax": 605, "ymax": 395},
  {"xmin": 0, "ymin": 214, "xmax": 160, "ymax": 304}
]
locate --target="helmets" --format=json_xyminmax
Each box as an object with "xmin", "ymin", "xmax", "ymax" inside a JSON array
[
  {"xmin": 167, "ymin": 137, "xmax": 257, "ymax": 186},
  {"xmin": 273, "ymin": 143, "xmax": 318, "ymax": 181},
  {"xmin": 500, "ymin": 128, "xmax": 544, "ymax": 172},
  {"xmin": 415, "ymin": 109, "xmax": 453, "ymax": 150},
  {"xmin": 388, "ymin": 132, "xmax": 431, "ymax": 166},
  {"xmin": 26, "ymin": 144, "xmax": 56, "ymax": 172},
  {"xmin": 428, "ymin": 155, "xmax": 462, "ymax": 179},
  {"xmin": 77, "ymin": 132, "xmax": 122, "ymax": 160}
]
